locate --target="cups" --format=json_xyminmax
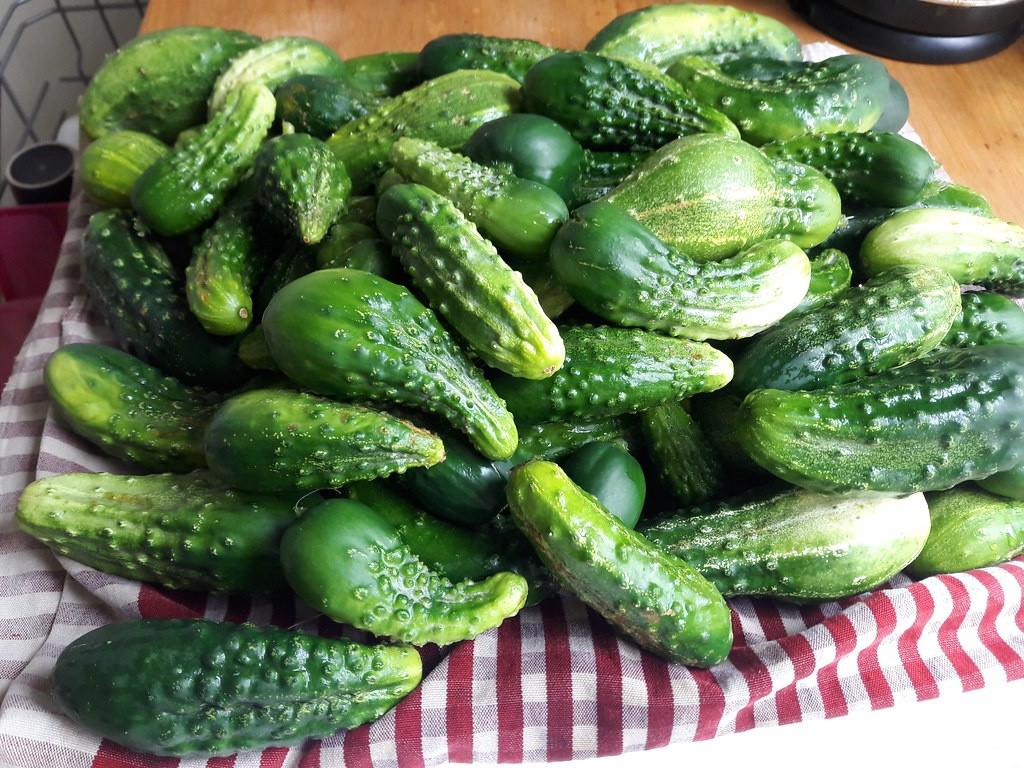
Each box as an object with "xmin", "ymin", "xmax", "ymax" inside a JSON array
[{"xmin": 4, "ymin": 143, "xmax": 74, "ymax": 204}]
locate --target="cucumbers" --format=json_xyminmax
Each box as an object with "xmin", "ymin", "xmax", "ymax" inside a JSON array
[{"xmin": 18, "ymin": 0, "xmax": 1024, "ymax": 757}]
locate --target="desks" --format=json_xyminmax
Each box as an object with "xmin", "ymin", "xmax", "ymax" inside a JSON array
[{"xmin": 0, "ymin": 0, "xmax": 1024, "ymax": 768}]
